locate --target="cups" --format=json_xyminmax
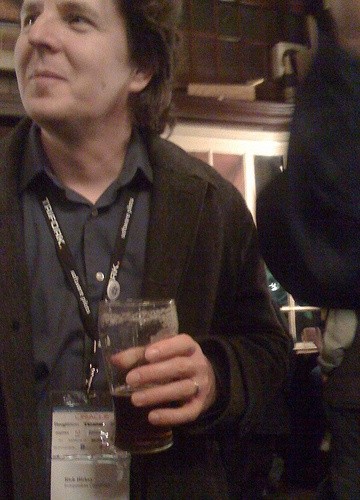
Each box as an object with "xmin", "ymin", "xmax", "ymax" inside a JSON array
[{"xmin": 98, "ymin": 298, "xmax": 173, "ymax": 455}]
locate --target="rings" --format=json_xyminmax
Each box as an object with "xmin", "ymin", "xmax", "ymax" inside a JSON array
[{"xmin": 190, "ymin": 378, "xmax": 199, "ymax": 399}]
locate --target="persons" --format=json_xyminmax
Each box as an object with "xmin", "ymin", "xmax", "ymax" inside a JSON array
[
  {"xmin": 1, "ymin": 0, "xmax": 294, "ymax": 500},
  {"xmin": 301, "ymin": 309, "xmax": 360, "ymax": 449}
]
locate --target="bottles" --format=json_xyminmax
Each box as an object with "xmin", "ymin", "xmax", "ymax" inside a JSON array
[{"xmin": 281, "ymin": 53, "xmax": 299, "ymax": 103}]
